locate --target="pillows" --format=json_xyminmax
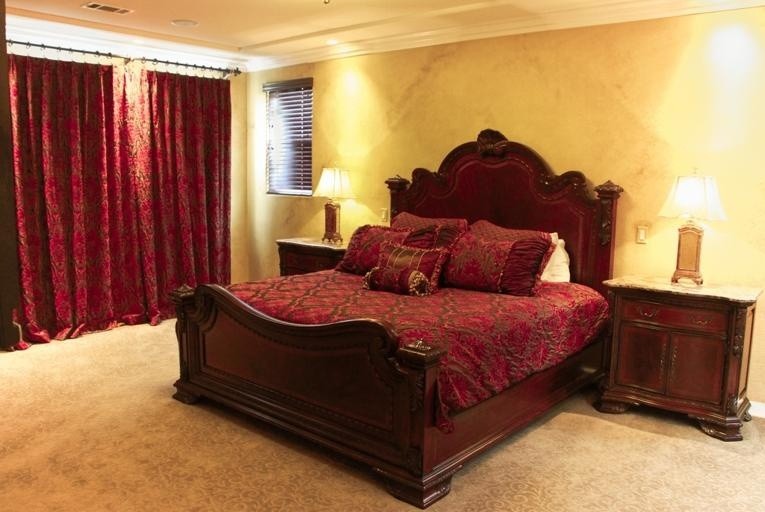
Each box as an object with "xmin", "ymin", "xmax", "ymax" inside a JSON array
[
  {"xmin": 468, "ymin": 220, "xmax": 551, "ymax": 248},
  {"xmin": 365, "ymin": 268, "xmax": 427, "ymax": 296},
  {"xmin": 441, "ymin": 233, "xmax": 547, "ymax": 295},
  {"xmin": 390, "ymin": 212, "xmax": 466, "ymax": 240},
  {"xmin": 335, "ymin": 225, "xmax": 459, "ymax": 288}
]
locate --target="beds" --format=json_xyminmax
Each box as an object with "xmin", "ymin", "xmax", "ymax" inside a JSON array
[{"xmin": 166, "ymin": 127, "xmax": 624, "ymax": 510}]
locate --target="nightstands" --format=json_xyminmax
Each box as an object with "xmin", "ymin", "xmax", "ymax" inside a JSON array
[
  {"xmin": 275, "ymin": 237, "xmax": 349, "ymax": 278},
  {"xmin": 598, "ymin": 275, "xmax": 761, "ymax": 443}
]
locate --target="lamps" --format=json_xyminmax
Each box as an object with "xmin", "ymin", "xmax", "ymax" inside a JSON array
[
  {"xmin": 656, "ymin": 172, "xmax": 727, "ymax": 286},
  {"xmin": 312, "ymin": 166, "xmax": 358, "ymax": 248}
]
